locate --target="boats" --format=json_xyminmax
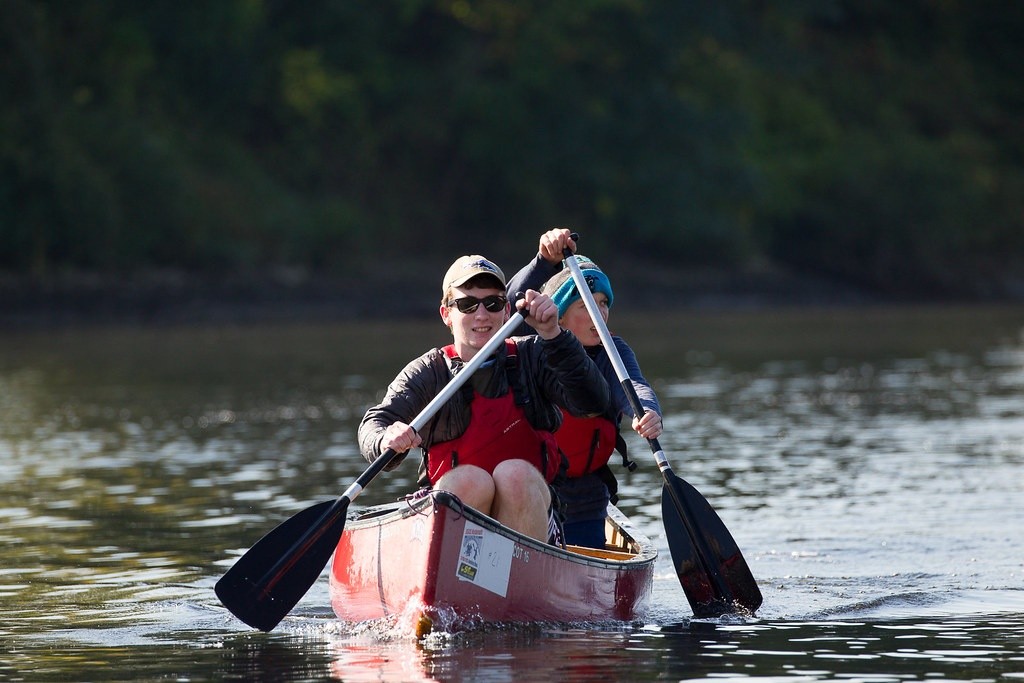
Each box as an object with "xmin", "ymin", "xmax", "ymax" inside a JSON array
[{"xmin": 334, "ymin": 490, "xmax": 658, "ymax": 645}]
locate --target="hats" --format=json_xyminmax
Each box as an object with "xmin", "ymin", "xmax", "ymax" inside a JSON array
[
  {"xmin": 541, "ymin": 254, "xmax": 613, "ymax": 324},
  {"xmin": 443, "ymin": 255, "xmax": 507, "ymax": 295}
]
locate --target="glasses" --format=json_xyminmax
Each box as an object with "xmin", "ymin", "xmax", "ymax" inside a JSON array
[{"xmin": 447, "ymin": 296, "xmax": 507, "ymax": 314}]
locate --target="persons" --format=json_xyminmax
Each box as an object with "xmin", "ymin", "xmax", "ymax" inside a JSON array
[
  {"xmin": 503, "ymin": 230, "xmax": 664, "ymax": 540},
  {"xmin": 357, "ymin": 254, "xmax": 617, "ymax": 547}
]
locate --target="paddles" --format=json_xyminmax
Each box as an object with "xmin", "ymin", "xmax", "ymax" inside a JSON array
[
  {"xmin": 560, "ymin": 232, "xmax": 764, "ymax": 620},
  {"xmin": 213, "ymin": 292, "xmax": 534, "ymax": 633}
]
